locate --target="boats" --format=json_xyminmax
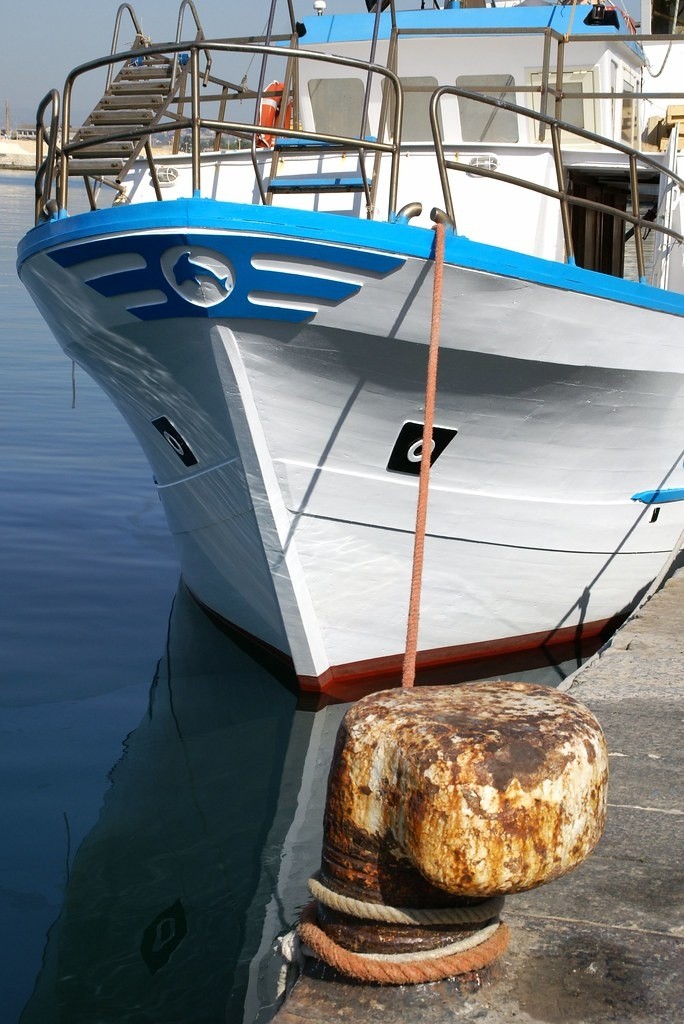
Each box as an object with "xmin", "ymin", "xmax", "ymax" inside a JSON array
[{"xmin": 16, "ymin": 2, "xmax": 682, "ymax": 698}]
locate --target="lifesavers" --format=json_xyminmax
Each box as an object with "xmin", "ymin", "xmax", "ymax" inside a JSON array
[{"xmin": 255, "ymin": 81, "xmax": 292, "ymax": 148}]
